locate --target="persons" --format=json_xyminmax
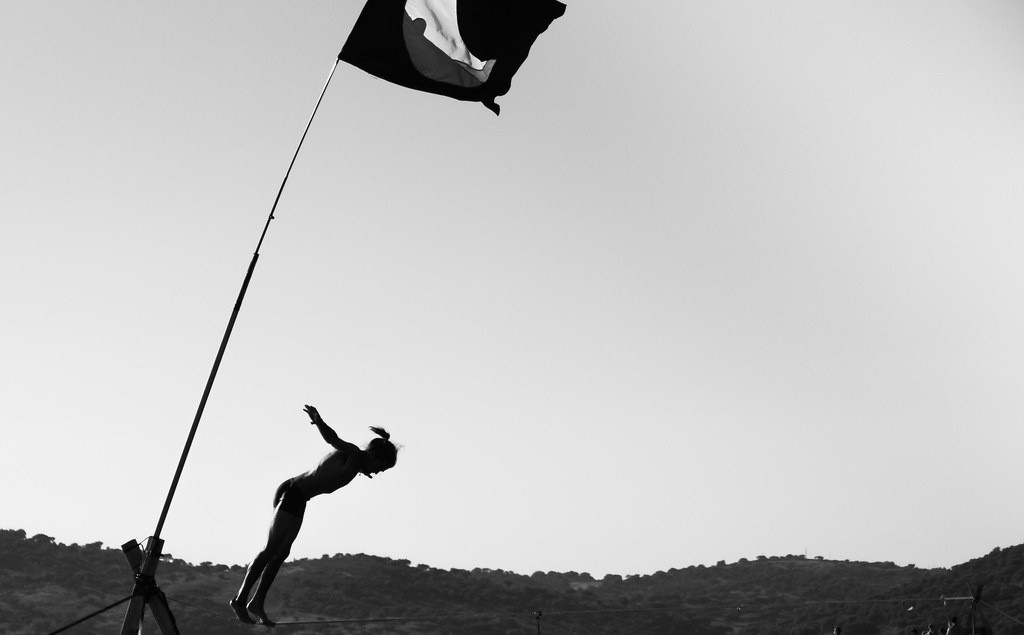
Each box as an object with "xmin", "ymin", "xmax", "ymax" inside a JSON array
[
  {"xmin": 229, "ymin": 404, "xmax": 397, "ymax": 628},
  {"xmin": 828, "ymin": 624, "xmax": 845, "ymax": 635},
  {"xmin": 910, "ymin": 615, "xmax": 965, "ymax": 635}
]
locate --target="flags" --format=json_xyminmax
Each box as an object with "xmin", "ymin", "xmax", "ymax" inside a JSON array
[{"xmin": 339, "ymin": 1, "xmax": 568, "ymax": 117}]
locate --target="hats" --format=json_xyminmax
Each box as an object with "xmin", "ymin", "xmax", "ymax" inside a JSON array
[
  {"xmin": 911, "ymin": 627, "xmax": 918, "ymax": 631},
  {"xmin": 951, "ymin": 617, "xmax": 957, "ymax": 623}
]
